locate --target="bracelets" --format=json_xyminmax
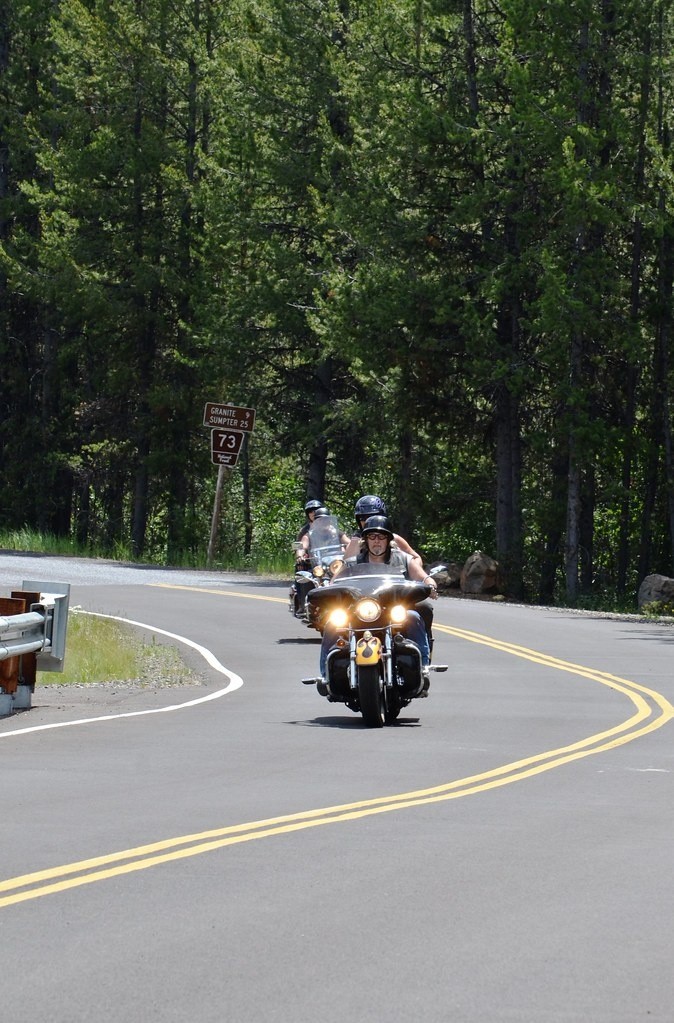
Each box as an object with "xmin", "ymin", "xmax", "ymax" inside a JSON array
[{"xmin": 429, "ymin": 584, "xmax": 437, "ymax": 592}]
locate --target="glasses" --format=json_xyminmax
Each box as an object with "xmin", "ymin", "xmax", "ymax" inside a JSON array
[
  {"xmin": 366, "ymin": 533, "xmax": 388, "ymax": 540},
  {"xmin": 359, "ymin": 516, "xmax": 368, "ymax": 521}
]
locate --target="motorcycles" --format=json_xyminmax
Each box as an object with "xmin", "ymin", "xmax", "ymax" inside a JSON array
[
  {"xmin": 288, "ymin": 514, "xmax": 348, "ymax": 637},
  {"xmin": 295, "ymin": 562, "xmax": 450, "ymax": 728}
]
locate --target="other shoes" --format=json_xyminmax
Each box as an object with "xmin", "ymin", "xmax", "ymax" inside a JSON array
[
  {"xmin": 316, "ymin": 682, "xmax": 329, "ymax": 696},
  {"xmin": 297, "ymin": 608, "xmax": 302, "ymax": 613},
  {"xmin": 415, "ymin": 677, "xmax": 430, "ymax": 698}
]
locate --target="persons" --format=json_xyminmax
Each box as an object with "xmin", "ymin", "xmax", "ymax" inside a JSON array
[
  {"xmin": 320, "ymin": 516, "xmax": 438, "ymax": 693},
  {"xmin": 343, "ymin": 495, "xmax": 433, "ymax": 664},
  {"xmin": 296, "ymin": 508, "xmax": 350, "ymax": 616},
  {"xmin": 293, "ymin": 500, "xmax": 322, "ymax": 616}
]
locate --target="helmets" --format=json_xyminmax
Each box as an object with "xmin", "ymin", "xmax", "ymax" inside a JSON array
[
  {"xmin": 314, "ymin": 507, "xmax": 330, "ymax": 517},
  {"xmin": 361, "ymin": 515, "xmax": 394, "ymax": 541},
  {"xmin": 304, "ymin": 500, "xmax": 323, "ymax": 510},
  {"xmin": 354, "ymin": 495, "xmax": 387, "ymax": 530}
]
